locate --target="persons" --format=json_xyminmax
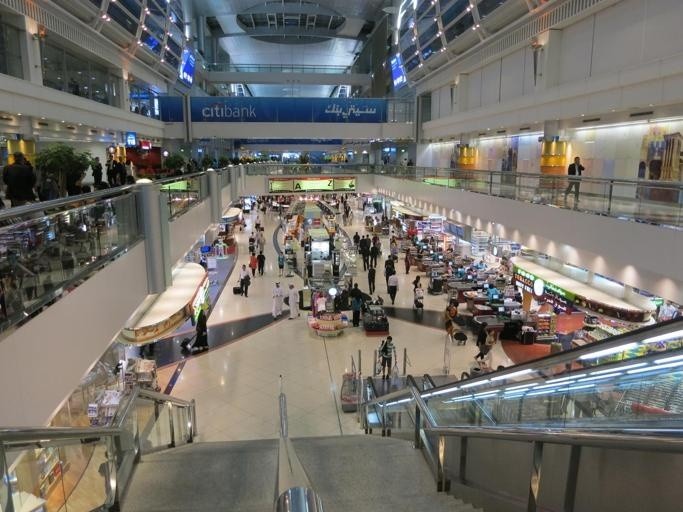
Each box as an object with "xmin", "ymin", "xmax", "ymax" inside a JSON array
[
  {"xmin": 272, "ymin": 282, "xmax": 368, "ymax": 327},
  {"xmin": 378, "ymin": 336, "xmax": 396, "ymax": 379},
  {"xmin": 450, "ymin": 159, "xmax": 456, "ymax": 178},
  {"xmin": 657, "ymin": 300, "xmax": 678, "ymax": 323},
  {"xmin": 353, "ymin": 231, "xmax": 399, "ymax": 304},
  {"xmin": 192, "ymin": 309, "xmax": 209, "ymax": 351},
  {"xmin": 383, "ymin": 152, "xmax": 413, "ymax": 174},
  {"xmin": 166, "ymin": 154, "xmax": 217, "ymax": 176},
  {"xmin": 408, "ymin": 227, "xmax": 517, "ymax": 310},
  {"xmin": 237, "ymin": 194, "xmax": 288, "ymax": 298},
  {"xmin": 0, "ymin": 152, "xmax": 37, "ymax": 211},
  {"xmin": 322, "ymin": 193, "xmax": 380, "ymax": 230},
  {"xmin": 445, "ymin": 303, "xmax": 496, "ymax": 368},
  {"xmin": 564, "ymin": 157, "xmax": 584, "ymax": 204},
  {"xmin": 89, "ymin": 153, "xmax": 127, "ymax": 190}
]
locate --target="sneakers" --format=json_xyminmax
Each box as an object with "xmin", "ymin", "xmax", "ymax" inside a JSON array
[{"xmin": 382, "ymin": 375, "xmax": 389, "ymax": 379}]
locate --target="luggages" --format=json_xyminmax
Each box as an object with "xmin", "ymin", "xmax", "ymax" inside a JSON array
[
  {"xmin": 180, "ymin": 334, "xmax": 197, "ymax": 348},
  {"xmin": 233, "ymin": 287, "xmax": 240, "ymax": 294}
]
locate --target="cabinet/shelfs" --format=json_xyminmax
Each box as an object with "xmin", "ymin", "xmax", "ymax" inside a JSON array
[
  {"xmin": 537, "ymin": 314, "xmax": 550, "ymax": 338},
  {"xmin": 309, "ymin": 310, "xmax": 349, "ymax": 337}
]
